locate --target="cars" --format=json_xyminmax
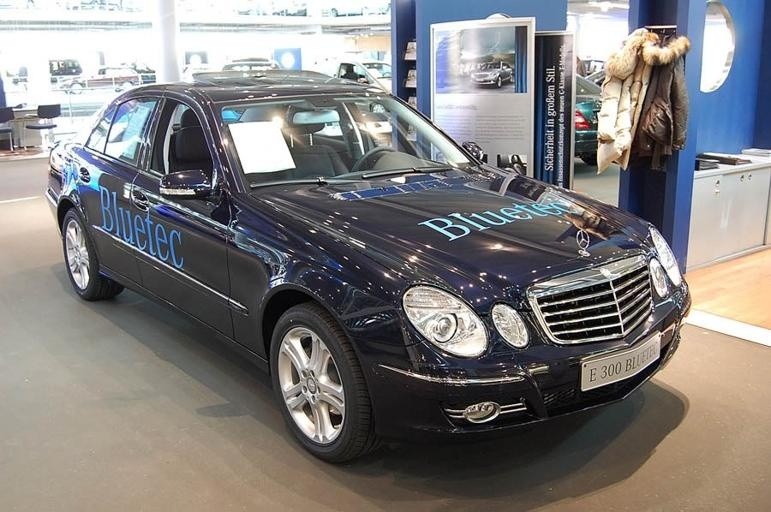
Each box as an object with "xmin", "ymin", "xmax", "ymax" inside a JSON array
[
  {"xmin": 573, "ymin": 69, "xmax": 606, "ymax": 166},
  {"xmin": 11, "ymin": 59, "xmax": 160, "ymax": 95},
  {"xmin": 582, "ymin": 56, "xmax": 607, "ymax": 76},
  {"xmin": 223, "ymin": 58, "xmax": 281, "ymax": 72},
  {"xmin": 46, "ymin": 69, "xmax": 691, "ymax": 467},
  {"xmin": 587, "ymin": 69, "xmax": 608, "ymax": 88},
  {"xmin": 471, "ymin": 62, "xmax": 516, "ymax": 89}
]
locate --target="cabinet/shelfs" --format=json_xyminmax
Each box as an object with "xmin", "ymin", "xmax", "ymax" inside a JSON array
[{"xmin": 686, "ymin": 167, "xmax": 771, "ymax": 272}]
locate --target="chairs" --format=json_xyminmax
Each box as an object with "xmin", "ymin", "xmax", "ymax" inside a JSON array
[
  {"xmin": 284, "ymin": 103, "xmax": 350, "ymax": 181},
  {"xmin": 171, "ymin": 108, "xmax": 216, "ymax": 185},
  {"xmin": 0, "ymin": 103, "xmax": 62, "ymax": 153}
]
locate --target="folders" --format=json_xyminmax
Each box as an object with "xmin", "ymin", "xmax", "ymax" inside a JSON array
[{"xmin": 695, "ymin": 152, "xmax": 751, "ymax": 170}]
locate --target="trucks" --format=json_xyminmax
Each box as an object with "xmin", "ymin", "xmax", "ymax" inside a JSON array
[{"xmin": 272, "ymin": 33, "xmax": 393, "ymax": 93}]
